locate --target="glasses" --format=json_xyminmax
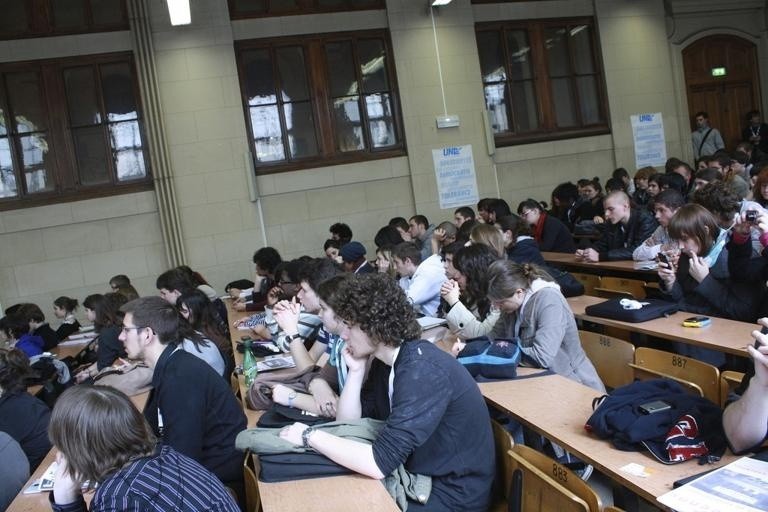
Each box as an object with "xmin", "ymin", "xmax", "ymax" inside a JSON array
[
  {"xmin": 279, "ymin": 278, "xmax": 296, "ymax": 284},
  {"xmin": 120, "ymin": 323, "xmax": 155, "ymax": 336}
]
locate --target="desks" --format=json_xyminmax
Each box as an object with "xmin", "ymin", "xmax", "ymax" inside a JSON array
[
  {"xmin": 529, "ymin": 250, "xmax": 668, "ymax": 296},
  {"xmin": 7, "ymin": 325, "xmax": 155, "ymax": 512},
  {"xmin": 222, "ymin": 296, "xmax": 767, "ymax": 512}
]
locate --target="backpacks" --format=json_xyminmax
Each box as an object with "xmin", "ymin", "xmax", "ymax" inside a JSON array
[
  {"xmin": 449, "ymin": 335, "xmax": 557, "ymax": 384},
  {"xmin": 254, "ymin": 400, "xmax": 368, "ymax": 483}
]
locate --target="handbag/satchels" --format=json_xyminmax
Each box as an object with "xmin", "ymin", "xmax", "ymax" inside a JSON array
[{"xmin": 585, "ymin": 289, "xmax": 681, "ymax": 323}]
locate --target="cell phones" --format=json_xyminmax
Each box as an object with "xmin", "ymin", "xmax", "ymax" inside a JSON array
[
  {"xmin": 684, "ymin": 315, "xmax": 711, "ymax": 328},
  {"xmin": 242, "ymin": 335, "xmax": 251, "ymax": 340},
  {"xmin": 658, "ymin": 253, "xmax": 671, "ymax": 269},
  {"xmin": 640, "ymin": 400, "xmax": 673, "ymax": 415}
]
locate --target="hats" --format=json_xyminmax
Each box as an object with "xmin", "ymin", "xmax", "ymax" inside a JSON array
[{"xmin": 339, "ymin": 241, "xmax": 366, "ymax": 261}]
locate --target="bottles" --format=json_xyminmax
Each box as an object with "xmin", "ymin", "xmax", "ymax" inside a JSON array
[{"xmin": 243, "ymin": 339, "xmax": 257, "ymax": 387}]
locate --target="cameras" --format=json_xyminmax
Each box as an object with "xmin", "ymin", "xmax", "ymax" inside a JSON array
[{"xmin": 747, "ymin": 211, "xmax": 758, "ymax": 221}]
